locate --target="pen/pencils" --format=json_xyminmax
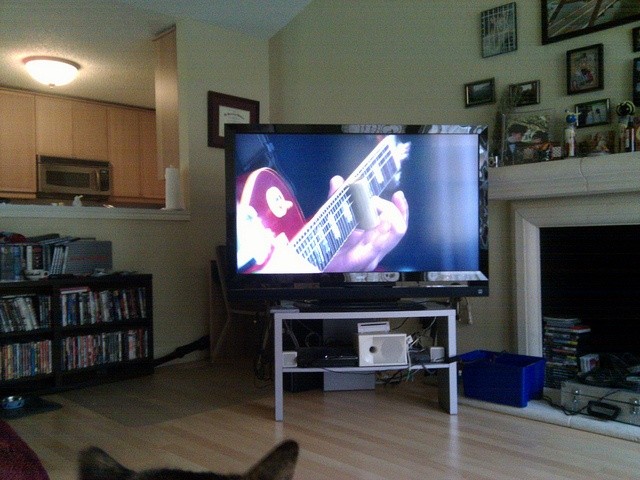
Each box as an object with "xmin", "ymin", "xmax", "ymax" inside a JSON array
[
  {"xmin": 630, "ymin": 121, "xmax": 636, "ymax": 151},
  {"xmin": 623, "ymin": 121, "xmax": 630, "ymax": 152}
]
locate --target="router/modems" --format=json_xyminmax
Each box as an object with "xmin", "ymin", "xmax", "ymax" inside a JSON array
[{"xmin": 355, "ymin": 320, "xmax": 393, "ymax": 335}]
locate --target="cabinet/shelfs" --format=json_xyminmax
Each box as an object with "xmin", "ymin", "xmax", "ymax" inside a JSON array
[{"xmin": 0, "ymin": 273, "xmax": 155, "ymax": 399}]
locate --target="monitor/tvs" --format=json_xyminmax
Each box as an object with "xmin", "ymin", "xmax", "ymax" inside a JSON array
[{"xmin": 223, "ymin": 120, "xmax": 489, "ymax": 312}]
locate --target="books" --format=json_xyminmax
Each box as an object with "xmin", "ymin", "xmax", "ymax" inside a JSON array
[
  {"xmin": 1, "ymin": 235, "xmax": 113, "ymax": 283},
  {"xmin": 543, "ymin": 315, "xmax": 590, "ymax": 385},
  {"xmin": 1, "ymin": 293, "xmax": 51, "ymax": 335},
  {"xmin": 58, "ymin": 286, "xmax": 148, "ymax": 328},
  {"xmin": 59, "ymin": 330, "xmax": 151, "ymax": 370},
  {"xmin": 1, "ymin": 339, "xmax": 52, "ymax": 381}
]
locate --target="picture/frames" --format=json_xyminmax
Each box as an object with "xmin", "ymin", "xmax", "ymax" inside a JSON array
[
  {"xmin": 631, "ymin": 27, "xmax": 640, "ymax": 53},
  {"xmin": 502, "ymin": 108, "xmax": 564, "ymax": 166},
  {"xmin": 480, "ymin": 1, "xmax": 517, "ymax": 59},
  {"xmin": 208, "ymin": 90, "xmax": 260, "ymax": 150},
  {"xmin": 633, "ymin": 57, "xmax": 640, "ymax": 107},
  {"xmin": 507, "ymin": 79, "xmax": 540, "ymax": 108},
  {"xmin": 566, "ymin": 43, "xmax": 604, "ymax": 95},
  {"xmin": 540, "ymin": 0, "xmax": 640, "ymax": 45},
  {"xmin": 575, "ymin": 98, "xmax": 610, "ymax": 129},
  {"xmin": 464, "ymin": 77, "xmax": 495, "ymax": 108}
]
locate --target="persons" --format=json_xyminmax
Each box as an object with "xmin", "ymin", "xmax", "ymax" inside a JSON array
[
  {"xmin": 574, "ymin": 53, "xmax": 593, "ymax": 82},
  {"xmin": 504, "ymin": 123, "xmax": 527, "ymax": 163},
  {"xmin": 533, "ymin": 131, "xmax": 551, "ymax": 151},
  {"xmin": 586, "ymin": 105, "xmax": 603, "ymax": 125}
]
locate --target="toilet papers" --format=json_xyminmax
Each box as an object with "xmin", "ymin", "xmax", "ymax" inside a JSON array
[{"xmin": 163, "ymin": 166, "xmax": 182, "ymax": 211}]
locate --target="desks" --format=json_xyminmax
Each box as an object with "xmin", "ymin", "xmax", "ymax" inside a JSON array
[{"xmin": 271, "ymin": 309, "xmax": 457, "ymax": 421}]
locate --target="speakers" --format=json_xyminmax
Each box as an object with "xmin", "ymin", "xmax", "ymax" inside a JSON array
[{"xmin": 354, "ymin": 334, "xmax": 410, "ymax": 368}]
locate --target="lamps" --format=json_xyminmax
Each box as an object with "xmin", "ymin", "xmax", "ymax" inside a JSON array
[{"xmin": 22, "ymin": 57, "xmax": 80, "ymax": 88}]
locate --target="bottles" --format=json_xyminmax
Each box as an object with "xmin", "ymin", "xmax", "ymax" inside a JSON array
[{"xmin": 164, "ymin": 164, "xmax": 184, "ymax": 209}]
